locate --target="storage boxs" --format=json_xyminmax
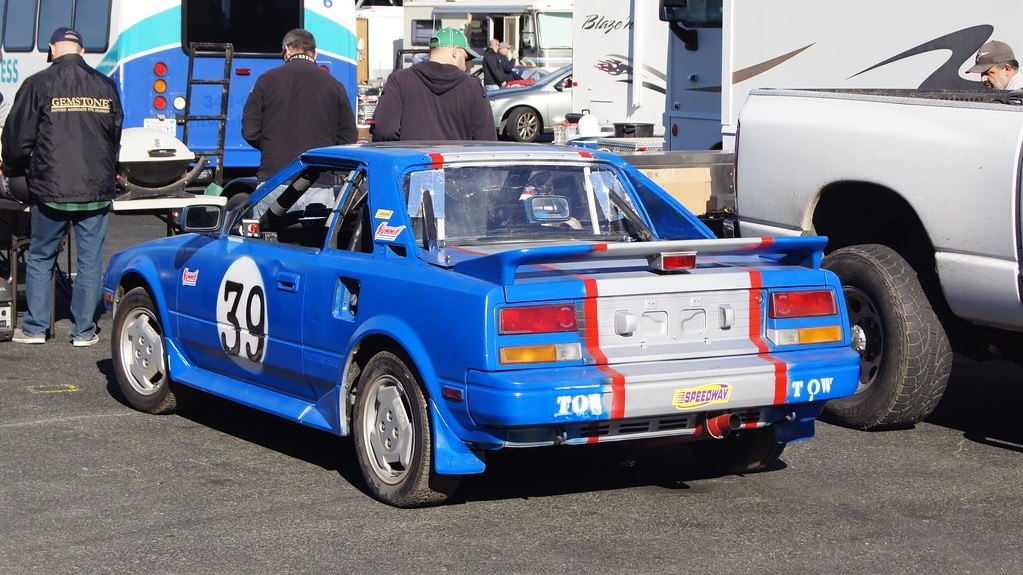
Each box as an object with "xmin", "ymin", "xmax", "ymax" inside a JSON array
[{"xmin": 115, "ymin": 126, "xmax": 197, "ymax": 188}]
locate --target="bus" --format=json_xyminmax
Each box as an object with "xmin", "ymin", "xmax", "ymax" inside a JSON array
[
  {"xmin": 0, "ymin": 0, "xmax": 358, "ymax": 184},
  {"xmin": 359, "ymin": 0, "xmax": 1023, "ymax": 149}
]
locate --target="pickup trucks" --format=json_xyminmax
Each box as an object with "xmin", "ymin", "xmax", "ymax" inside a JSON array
[{"xmin": 720, "ymin": 87, "xmax": 1023, "ymax": 431}]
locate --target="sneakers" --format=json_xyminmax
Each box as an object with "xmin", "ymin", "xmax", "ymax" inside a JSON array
[
  {"xmin": 74, "ymin": 334, "xmax": 100, "ymax": 346},
  {"xmin": 11, "ymin": 327, "xmax": 45, "ymax": 343}
]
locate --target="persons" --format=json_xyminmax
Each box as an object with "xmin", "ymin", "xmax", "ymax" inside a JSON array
[
  {"xmin": 241, "ymin": 29, "xmax": 360, "ymax": 220},
  {"xmin": 964, "ymin": 40, "xmax": 1023, "ymax": 90},
  {"xmin": 483, "ymin": 39, "xmax": 518, "ymax": 92},
  {"xmin": 0, "ymin": 28, "xmax": 125, "ymax": 347},
  {"xmin": 369, "ymin": 27, "xmax": 499, "ymax": 240}
]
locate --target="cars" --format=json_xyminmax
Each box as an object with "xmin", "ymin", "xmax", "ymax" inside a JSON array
[
  {"xmin": 102, "ymin": 140, "xmax": 861, "ymax": 509},
  {"xmin": 487, "ymin": 63, "xmax": 573, "ymax": 142}
]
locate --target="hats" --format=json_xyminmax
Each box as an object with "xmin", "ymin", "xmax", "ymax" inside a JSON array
[
  {"xmin": 428, "ymin": 28, "xmax": 482, "ymax": 59},
  {"xmin": 965, "ymin": 41, "xmax": 1015, "ymax": 73},
  {"xmin": 47, "ymin": 28, "xmax": 83, "ymax": 63},
  {"xmin": 500, "ymin": 42, "xmax": 512, "ymax": 49}
]
